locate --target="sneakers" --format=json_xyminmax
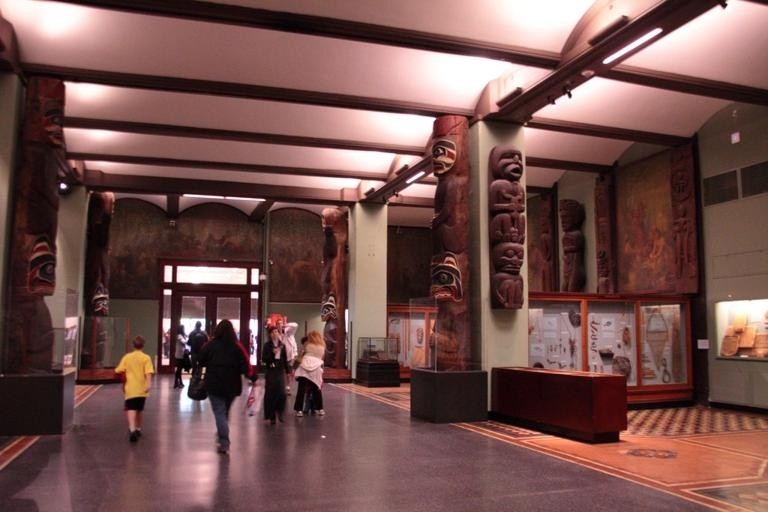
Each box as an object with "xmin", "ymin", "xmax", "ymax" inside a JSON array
[
  {"xmin": 136, "ymin": 429, "xmax": 142, "ymax": 437},
  {"xmin": 128, "ymin": 429, "xmax": 137, "ymax": 443},
  {"xmin": 287, "ymin": 389, "xmax": 291, "ymax": 396},
  {"xmin": 269, "ymin": 408, "xmax": 326, "ymax": 425},
  {"xmin": 217, "ymin": 444, "xmax": 229, "ymax": 453}
]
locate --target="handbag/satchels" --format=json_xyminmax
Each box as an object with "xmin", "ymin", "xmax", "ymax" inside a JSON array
[
  {"xmin": 184, "ymin": 350, "xmax": 189, "ymax": 359},
  {"xmin": 187, "ymin": 365, "xmax": 210, "ymax": 401}
]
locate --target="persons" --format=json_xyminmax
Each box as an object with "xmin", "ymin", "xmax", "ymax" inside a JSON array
[
  {"xmin": 163, "ymin": 328, "xmax": 170, "ymax": 358},
  {"xmin": 196, "ymin": 317, "xmax": 259, "ymax": 456},
  {"xmin": 300, "ymin": 335, "xmax": 318, "ymax": 416},
  {"xmin": 672, "ymin": 203, "xmax": 689, "ymax": 265},
  {"xmin": 173, "ymin": 325, "xmax": 187, "ymax": 389},
  {"xmin": 186, "ymin": 321, "xmax": 208, "ymax": 379},
  {"xmin": 620, "ymin": 197, "xmax": 672, "ymax": 288},
  {"xmin": 292, "ymin": 331, "xmax": 327, "ymax": 417},
  {"xmin": 486, "ymin": 240, "xmax": 526, "ymax": 310},
  {"xmin": 260, "ymin": 325, "xmax": 291, "ymax": 427},
  {"xmin": 536, "ymin": 202, "xmax": 556, "ymax": 292},
  {"xmin": 557, "ymin": 198, "xmax": 586, "ymax": 292},
  {"xmin": 488, "ymin": 144, "xmax": 527, "ymax": 244},
  {"xmin": 273, "ymin": 317, "xmax": 299, "ymax": 396},
  {"xmin": 113, "ymin": 336, "xmax": 154, "ymax": 443}
]
